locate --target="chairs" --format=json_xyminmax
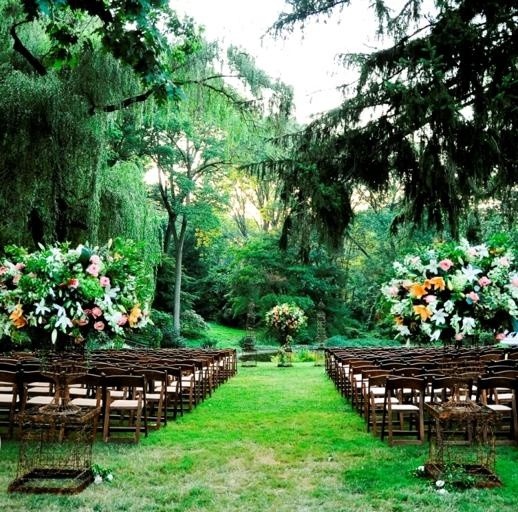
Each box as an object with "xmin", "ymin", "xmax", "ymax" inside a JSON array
[
  {"xmin": 1, "ymin": 347, "xmax": 238, "ymax": 445},
  {"xmin": 323, "ymin": 346, "xmax": 516, "ymax": 447}
]
